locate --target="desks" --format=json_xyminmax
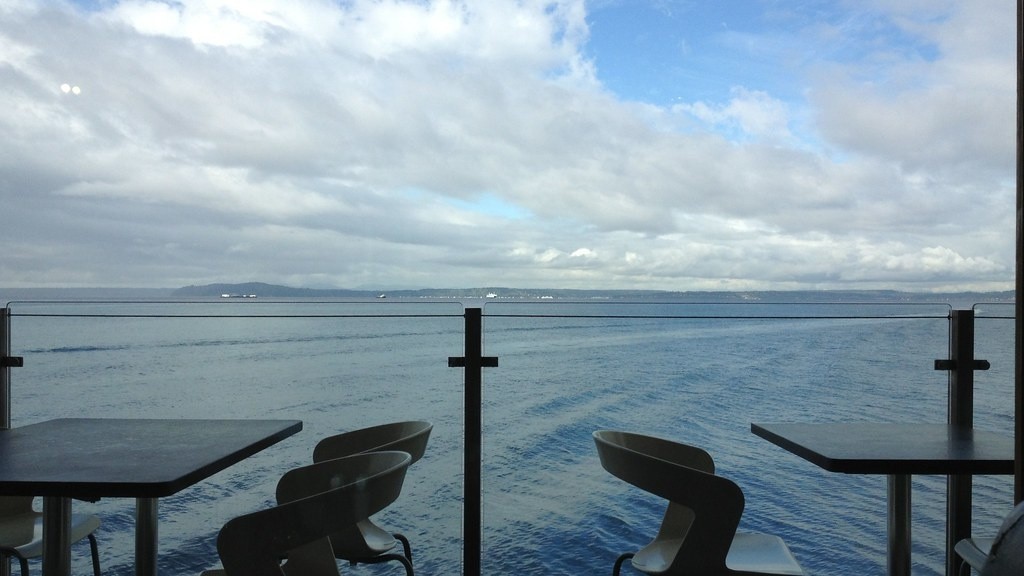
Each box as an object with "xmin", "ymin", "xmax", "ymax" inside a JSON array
[
  {"xmin": 0, "ymin": 417, "xmax": 304, "ymax": 576},
  {"xmin": 750, "ymin": 422, "xmax": 1015, "ymax": 576}
]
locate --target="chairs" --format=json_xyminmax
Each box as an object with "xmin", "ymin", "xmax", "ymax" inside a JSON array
[
  {"xmin": 954, "ymin": 501, "xmax": 1024, "ymax": 576},
  {"xmin": 592, "ymin": 429, "xmax": 806, "ymax": 576},
  {"xmin": 0, "ymin": 427, "xmax": 101, "ymax": 576},
  {"xmin": 200, "ymin": 449, "xmax": 413, "ymax": 576},
  {"xmin": 312, "ymin": 421, "xmax": 434, "ymax": 576}
]
